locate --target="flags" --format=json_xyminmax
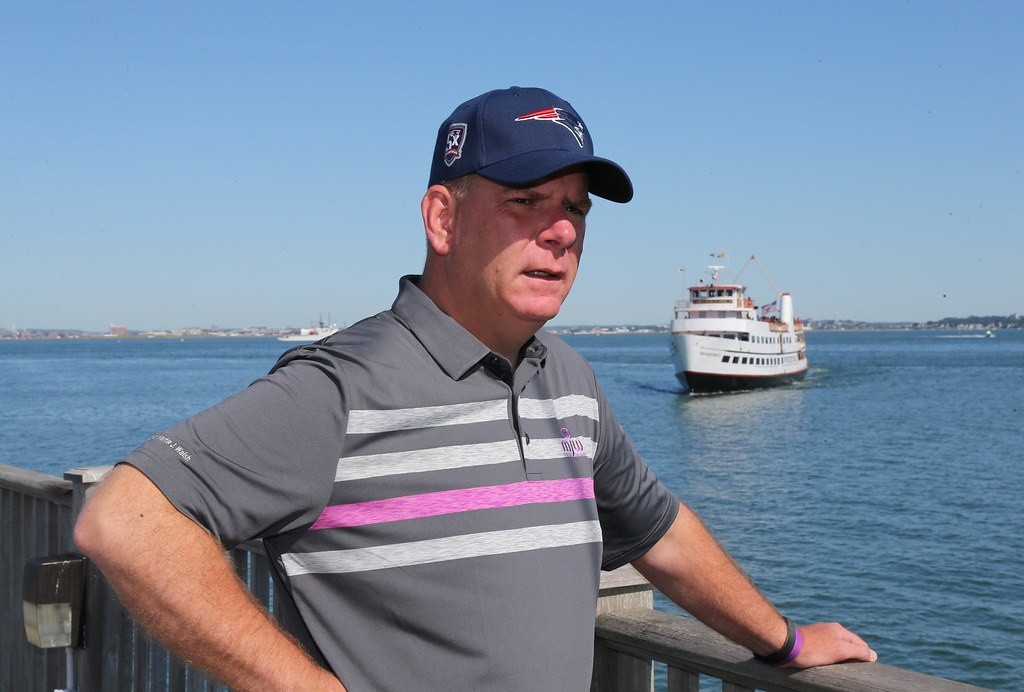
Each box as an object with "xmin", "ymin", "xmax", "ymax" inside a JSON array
[
  {"xmin": 748, "ymin": 255, "xmax": 757, "ymax": 260},
  {"xmin": 708, "ymin": 252, "xmax": 725, "ymax": 259},
  {"xmin": 677, "ymin": 267, "xmax": 684, "ymax": 272}
]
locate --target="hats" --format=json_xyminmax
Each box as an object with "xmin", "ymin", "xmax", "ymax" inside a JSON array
[{"xmin": 427, "ymin": 86, "xmax": 633, "ymax": 204}]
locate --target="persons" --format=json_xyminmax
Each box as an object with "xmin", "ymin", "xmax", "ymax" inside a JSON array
[{"xmin": 73, "ymin": 85, "xmax": 877, "ymax": 692}]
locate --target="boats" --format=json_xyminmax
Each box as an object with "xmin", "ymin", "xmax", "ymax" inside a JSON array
[
  {"xmin": 665, "ymin": 254, "xmax": 810, "ymax": 394},
  {"xmin": 277, "ymin": 314, "xmax": 341, "ymax": 342}
]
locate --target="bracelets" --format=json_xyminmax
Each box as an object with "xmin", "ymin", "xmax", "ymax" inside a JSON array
[{"xmin": 753, "ymin": 615, "xmax": 804, "ymax": 668}]
